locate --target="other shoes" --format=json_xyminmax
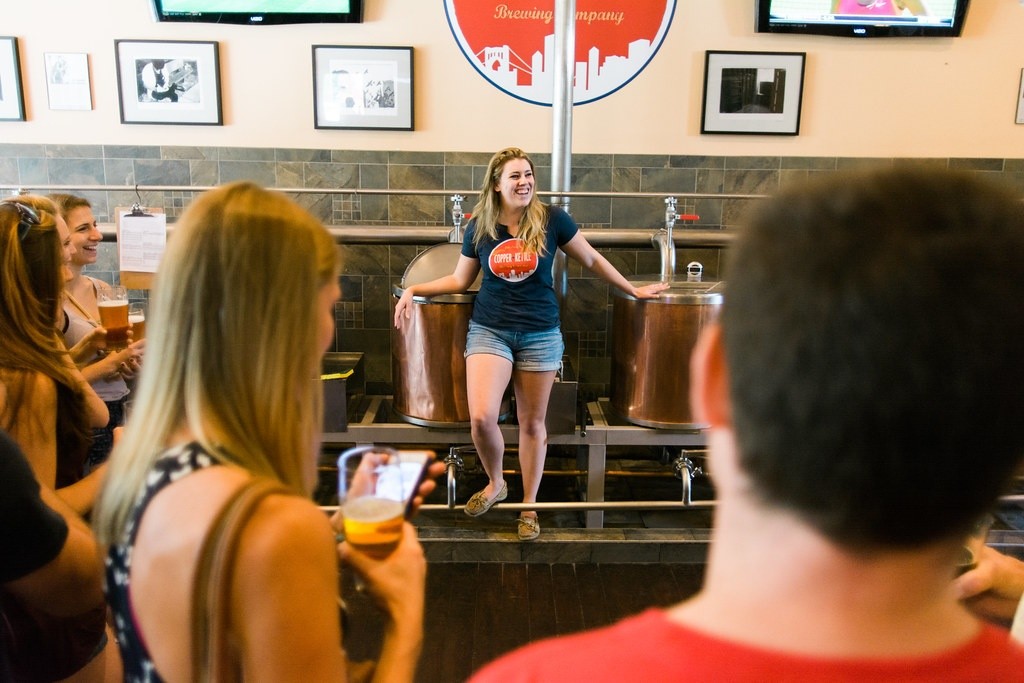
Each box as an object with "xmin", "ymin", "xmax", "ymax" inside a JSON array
[
  {"xmin": 517, "ymin": 510, "xmax": 540, "ymax": 540},
  {"xmin": 465, "ymin": 479, "xmax": 508, "ymax": 517}
]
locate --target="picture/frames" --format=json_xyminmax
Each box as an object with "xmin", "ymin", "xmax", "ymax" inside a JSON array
[
  {"xmin": 700, "ymin": 50, "xmax": 807, "ymax": 137},
  {"xmin": 0, "ymin": 36, "xmax": 27, "ymax": 121},
  {"xmin": 1015, "ymin": 68, "xmax": 1024, "ymax": 124},
  {"xmin": 312, "ymin": 45, "xmax": 414, "ymax": 131},
  {"xmin": 114, "ymin": 39, "xmax": 224, "ymax": 126}
]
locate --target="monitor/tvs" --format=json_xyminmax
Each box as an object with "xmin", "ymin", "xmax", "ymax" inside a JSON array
[
  {"xmin": 151, "ymin": 0, "xmax": 361, "ymax": 25},
  {"xmin": 755, "ymin": 0, "xmax": 968, "ymax": 37}
]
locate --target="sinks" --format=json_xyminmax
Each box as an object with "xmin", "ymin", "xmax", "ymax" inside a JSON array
[{"xmin": 627, "ymin": 280, "xmax": 722, "ymax": 295}]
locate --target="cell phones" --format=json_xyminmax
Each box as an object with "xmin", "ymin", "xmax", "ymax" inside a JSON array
[{"xmin": 373, "ymin": 451, "xmax": 432, "ymax": 519}]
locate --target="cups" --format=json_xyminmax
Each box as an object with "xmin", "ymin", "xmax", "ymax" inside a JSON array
[
  {"xmin": 97, "ymin": 287, "xmax": 129, "ymax": 351},
  {"xmin": 338, "ymin": 447, "xmax": 406, "ymax": 616},
  {"xmin": 128, "ymin": 302, "xmax": 147, "ymax": 344}
]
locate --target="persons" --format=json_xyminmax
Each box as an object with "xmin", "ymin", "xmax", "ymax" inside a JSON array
[
  {"xmin": 949, "ymin": 537, "xmax": 1024, "ymax": 640},
  {"xmin": 462, "ymin": 163, "xmax": 1024, "ymax": 683},
  {"xmin": 97, "ymin": 182, "xmax": 448, "ymax": 683},
  {"xmin": 394, "ymin": 148, "xmax": 670, "ymax": 541},
  {"xmin": 0, "ymin": 194, "xmax": 147, "ymax": 683},
  {"xmin": 142, "ymin": 59, "xmax": 178, "ymax": 102}
]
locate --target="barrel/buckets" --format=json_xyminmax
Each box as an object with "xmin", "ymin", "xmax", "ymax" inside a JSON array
[
  {"xmin": 389, "ymin": 283, "xmax": 512, "ymax": 430},
  {"xmin": 608, "ymin": 280, "xmax": 726, "ymax": 431}
]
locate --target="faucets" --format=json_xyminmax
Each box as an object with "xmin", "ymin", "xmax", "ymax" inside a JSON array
[
  {"xmin": 450, "ymin": 194, "xmax": 466, "ymax": 242},
  {"xmin": 664, "ymin": 197, "xmax": 700, "ymax": 250},
  {"xmin": 672, "ymin": 450, "xmax": 716, "ymax": 504},
  {"xmin": 441, "ymin": 444, "xmax": 476, "ymax": 510}
]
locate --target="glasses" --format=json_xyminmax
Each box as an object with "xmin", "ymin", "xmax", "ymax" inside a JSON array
[{"xmin": 0, "ymin": 200, "xmax": 39, "ymax": 241}]
